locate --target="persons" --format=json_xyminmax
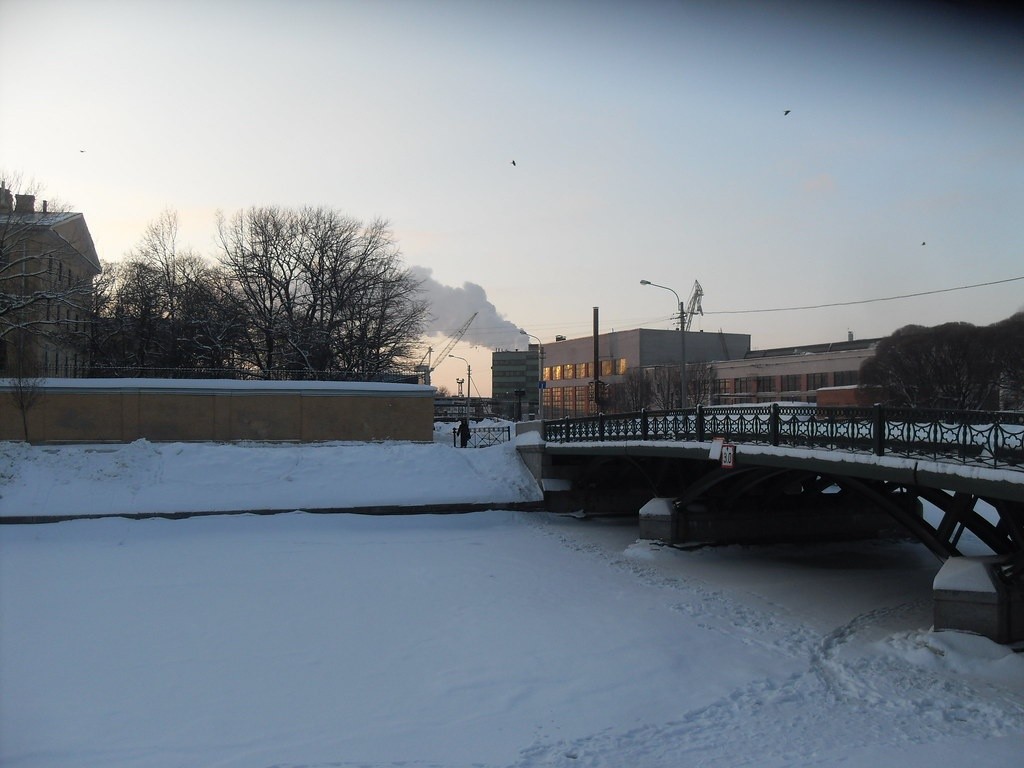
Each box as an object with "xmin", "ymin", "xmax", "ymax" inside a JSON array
[{"xmin": 457, "ymin": 418, "xmax": 471, "ymax": 448}]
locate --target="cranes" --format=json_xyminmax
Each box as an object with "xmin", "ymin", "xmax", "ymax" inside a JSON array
[
  {"xmin": 686, "ymin": 280, "xmax": 704, "ymax": 332},
  {"xmin": 414, "ymin": 311, "xmax": 482, "ymax": 385}
]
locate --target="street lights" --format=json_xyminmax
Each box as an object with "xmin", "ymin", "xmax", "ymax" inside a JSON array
[
  {"xmin": 640, "ymin": 279, "xmax": 687, "ymax": 409},
  {"xmin": 448, "ymin": 355, "xmax": 471, "ymax": 419},
  {"xmin": 520, "ymin": 329, "xmax": 543, "ymax": 419}
]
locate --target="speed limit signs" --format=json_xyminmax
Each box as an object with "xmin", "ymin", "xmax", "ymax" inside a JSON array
[{"xmin": 721, "ymin": 445, "xmax": 735, "ymax": 468}]
